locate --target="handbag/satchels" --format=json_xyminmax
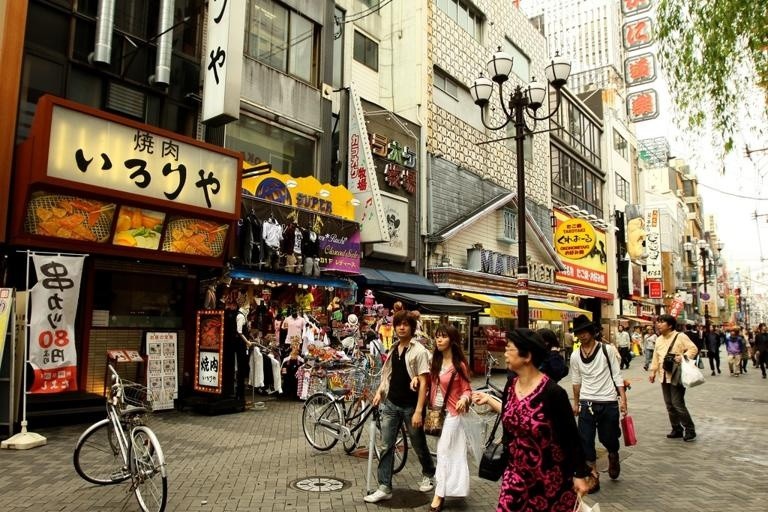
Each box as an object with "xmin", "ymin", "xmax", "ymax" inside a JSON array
[
  {"xmin": 613, "ymin": 377, "xmax": 630, "ymax": 396},
  {"xmin": 380, "ymin": 352, "xmax": 389, "ymax": 363},
  {"xmin": 620, "ymin": 413, "xmax": 638, "ymax": 449},
  {"xmin": 664, "ymin": 350, "xmax": 680, "ymax": 372},
  {"xmin": 478, "ymin": 436, "xmax": 510, "ymax": 482},
  {"xmin": 422, "ymin": 405, "xmax": 446, "ymax": 437}
]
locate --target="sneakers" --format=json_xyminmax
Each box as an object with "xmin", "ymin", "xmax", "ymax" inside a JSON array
[
  {"xmin": 619, "ymin": 361, "xmax": 651, "ymax": 371},
  {"xmin": 666, "ymin": 428, "xmax": 685, "ymax": 438},
  {"xmin": 683, "ymin": 429, "xmax": 697, "ymax": 443},
  {"xmin": 429, "ymin": 493, "xmax": 445, "ymax": 512},
  {"xmin": 363, "ymin": 485, "xmax": 395, "ymax": 503},
  {"xmin": 606, "ymin": 452, "xmax": 621, "ymax": 479},
  {"xmin": 583, "ymin": 469, "xmax": 601, "ymax": 495},
  {"xmin": 418, "ymin": 475, "xmax": 436, "ymax": 493},
  {"xmin": 709, "ymin": 360, "xmax": 768, "ymax": 379}
]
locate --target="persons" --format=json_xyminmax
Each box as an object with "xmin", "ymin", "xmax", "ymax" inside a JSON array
[
  {"xmin": 409, "ymin": 324, "xmax": 474, "ymax": 511},
  {"xmin": 647, "ymin": 313, "xmax": 700, "ymax": 442},
  {"xmin": 564, "ymin": 328, "xmax": 579, "ymax": 365},
  {"xmin": 244, "ymin": 208, "xmax": 320, "ymax": 278},
  {"xmin": 235, "ymin": 307, "xmax": 253, "ymax": 400},
  {"xmin": 571, "ymin": 314, "xmax": 628, "ymax": 495},
  {"xmin": 279, "ymin": 307, "xmax": 306, "ymax": 355},
  {"xmin": 614, "ymin": 322, "xmax": 768, "ymax": 380},
  {"xmin": 471, "ymin": 327, "xmax": 593, "ymax": 511},
  {"xmin": 536, "ymin": 327, "xmax": 569, "ymax": 383},
  {"xmin": 362, "ymin": 309, "xmax": 436, "ymax": 503}
]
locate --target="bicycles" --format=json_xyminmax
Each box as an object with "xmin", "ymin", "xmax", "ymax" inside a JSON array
[
  {"xmin": 299, "ymin": 330, "xmax": 504, "ymax": 473},
  {"xmin": 73, "ymin": 363, "xmax": 168, "ymax": 512}
]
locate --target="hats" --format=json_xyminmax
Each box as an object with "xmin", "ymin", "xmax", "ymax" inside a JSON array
[
  {"xmin": 569, "ymin": 314, "xmax": 597, "ymax": 335},
  {"xmin": 347, "ymin": 314, "xmax": 358, "ymax": 325}
]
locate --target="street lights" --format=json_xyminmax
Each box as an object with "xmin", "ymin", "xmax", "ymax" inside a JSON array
[
  {"xmin": 467, "ymin": 46, "xmax": 574, "ymax": 327},
  {"xmin": 683, "ymin": 236, "xmax": 725, "ymax": 330},
  {"xmin": 733, "ymin": 285, "xmax": 752, "ymax": 330}
]
